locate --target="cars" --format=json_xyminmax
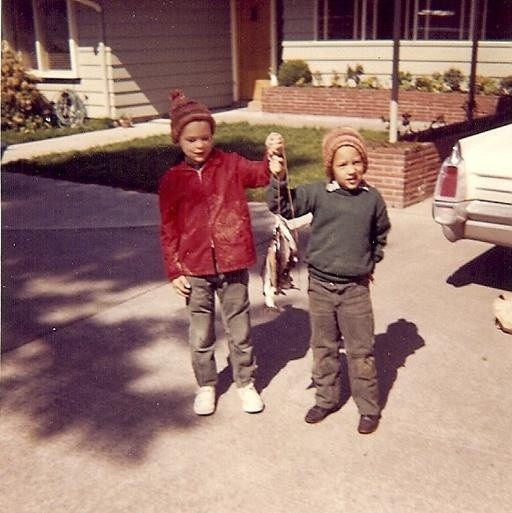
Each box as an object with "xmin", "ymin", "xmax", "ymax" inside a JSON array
[{"xmin": 431, "ymin": 123, "xmax": 512, "ymax": 250}]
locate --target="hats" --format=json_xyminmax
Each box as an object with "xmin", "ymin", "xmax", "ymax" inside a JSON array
[
  {"xmin": 168, "ymin": 88, "xmax": 215, "ymax": 143},
  {"xmin": 323, "ymin": 127, "xmax": 368, "ymax": 177}
]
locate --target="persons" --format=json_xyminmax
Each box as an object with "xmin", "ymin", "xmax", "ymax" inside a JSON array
[
  {"xmin": 159, "ymin": 89, "xmax": 287, "ymax": 416},
  {"xmin": 263, "ymin": 126, "xmax": 392, "ymax": 435}
]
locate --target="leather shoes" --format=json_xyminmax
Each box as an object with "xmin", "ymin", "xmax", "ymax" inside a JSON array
[
  {"xmin": 305, "ymin": 405, "xmax": 330, "ymax": 423},
  {"xmin": 358, "ymin": 414, "xmax": 378, "ymax": 434}
]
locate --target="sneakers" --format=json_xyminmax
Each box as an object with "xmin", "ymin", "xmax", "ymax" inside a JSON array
[
  {"xmin": 237, "ymin": 385, "xmax": 264, "ymax": 413},
  {"xmin": 194, "ymin": 386, "xmax": 216, "ymax": 415},
  {"xmin": 492, "ymin": 295, "xmax": 512, "ymax": 331}
]
